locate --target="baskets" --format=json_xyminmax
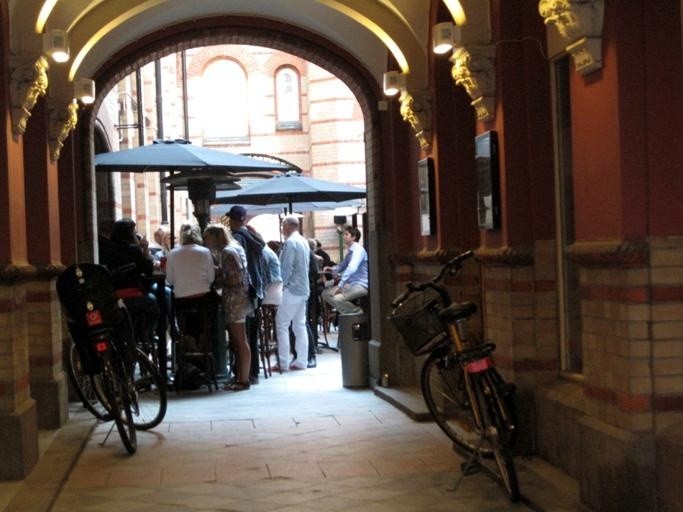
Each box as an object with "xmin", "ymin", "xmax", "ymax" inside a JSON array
[{"xmin": 389, "ymin": 288, "xmax": 446, "ymax": 357}]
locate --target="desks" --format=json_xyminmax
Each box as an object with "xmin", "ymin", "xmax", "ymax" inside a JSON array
[
  {"xmin": 317, "ymin": 270, "xmax": 336, "ymax": 347},
  {"xmin": 142, "ymin": 271, "xmax": 166, "ymax": 376}
]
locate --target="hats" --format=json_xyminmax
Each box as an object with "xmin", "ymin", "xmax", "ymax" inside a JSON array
[{"xmin": 225, "ymin": 206, "xmax": 247, "ymax": 221}]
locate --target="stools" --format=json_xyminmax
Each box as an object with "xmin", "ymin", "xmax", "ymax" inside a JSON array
[
  {"xmin": 350, "ymin": 295, "xmax": 370, "ymax": 313},
  {"xmin": 111, "ymin": 261, "xmax": 158, "ymax": 392},
  {"xmin": 258, "ymin": 303, "xmax": 282, "ymax": 379},
  {"xmin": 168, "ymin": 283, "xmax": 220, "ymax": 394}
]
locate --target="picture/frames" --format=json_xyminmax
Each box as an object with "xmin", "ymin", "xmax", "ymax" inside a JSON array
[
  {"xmin": 416, "ymin": 156, "xmax": 435, "ymax": 236},
  {"xmin": 474, "ymin": 129, "xmax": 501, "ymax": 232}
]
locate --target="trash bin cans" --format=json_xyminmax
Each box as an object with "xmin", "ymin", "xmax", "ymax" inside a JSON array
[{"xmin": 339, "ymin": 314, "xmax": 368, "ymax": 388}]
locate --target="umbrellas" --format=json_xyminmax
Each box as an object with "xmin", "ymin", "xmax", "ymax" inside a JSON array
[
  {"xmin": 209, "ymin": 174, "xmax": 366, "ymax": 213},
  {"xmin": 95, "ymin": 137, "xmax": 291, "ymax": 250},
  {"xmin": 207, "ymin": 200, "xmax": 370, "ymax": 216}
]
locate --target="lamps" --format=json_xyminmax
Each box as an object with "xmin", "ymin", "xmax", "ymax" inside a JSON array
[
  {"xmin": 431, "ymin": 21, "xmax": 454, "ymax": 56},
  {"xmin": 72, "ymin": 78, "xmax": 96, "ymax": 104},
  {"xmin": 382, "ymin": 70, "xmax": 400, "ymax": 96},
  {"xmin": 42, "ymin": 28, "xmax": 70, "ymax": 63}
]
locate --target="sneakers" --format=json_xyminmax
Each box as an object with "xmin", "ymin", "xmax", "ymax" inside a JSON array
[{"xmin": 225, "ymin": 357, "xmax": 316, "ymax": 391}]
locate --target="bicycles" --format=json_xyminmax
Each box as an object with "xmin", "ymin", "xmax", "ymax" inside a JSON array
[
  {"xmin": 54, "ymin": 260, "xmax": 169, "ymax": 454},
  {"xmin": 380, "ymin": 241, "xmax": 528, "ymax": 506}
]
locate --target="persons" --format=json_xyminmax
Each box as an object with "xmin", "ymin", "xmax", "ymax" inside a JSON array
[
  {"xmin": 109, "ymin": 204, "xmax": 331, "ymax": 392},
  {"xmin": 321, "ymin": 225, "xmax": 368, "ymax": 316}
]
